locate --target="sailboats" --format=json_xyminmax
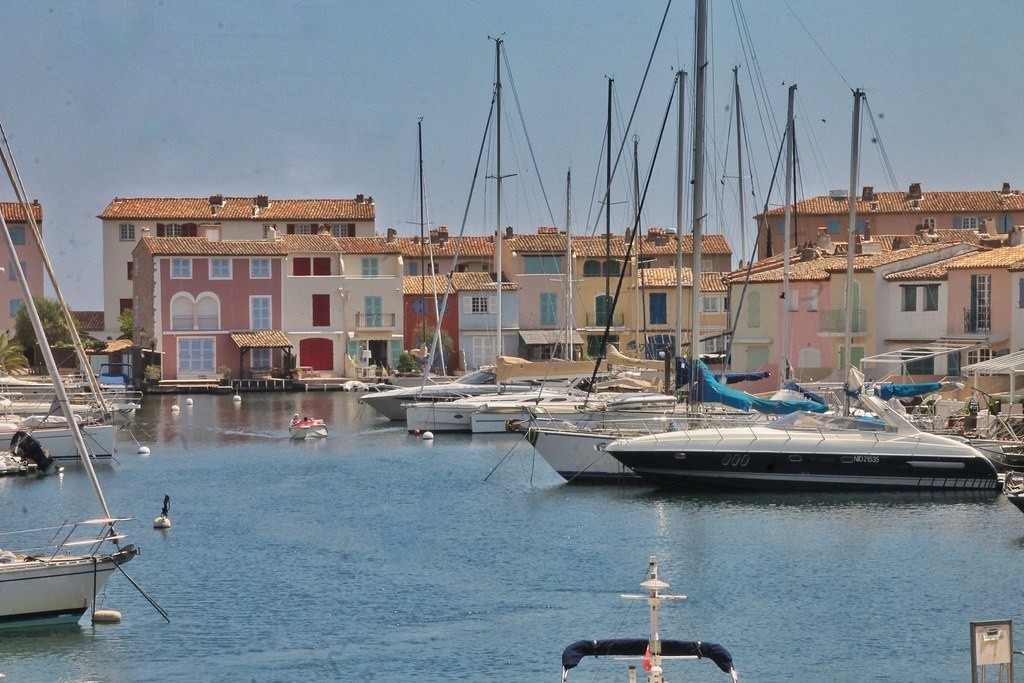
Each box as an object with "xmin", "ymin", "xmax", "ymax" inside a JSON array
[
  {"xmin": 0, "ymin": 208, "xmax": 142, "ymax": 626},
  {"xmin": 0, "ymin": 124, "xmax": 141, "ymax": 477},
  {"xmin": 358, "ymin": 0, "xmax": 797, "ymax": 486},
  {"xmin": 613, "ymin": 86, "xmax": 1008, "ymax": 490}
]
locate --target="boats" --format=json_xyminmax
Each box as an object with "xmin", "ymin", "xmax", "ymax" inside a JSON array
[{"xmin": 289, "ymin": 421, "xmax": 327, "ymax": 440}]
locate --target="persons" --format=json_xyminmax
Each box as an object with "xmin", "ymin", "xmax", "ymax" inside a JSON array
[{"xmin": 290, "ymin": 413, "xmax": 314, "ymax": 427}]
